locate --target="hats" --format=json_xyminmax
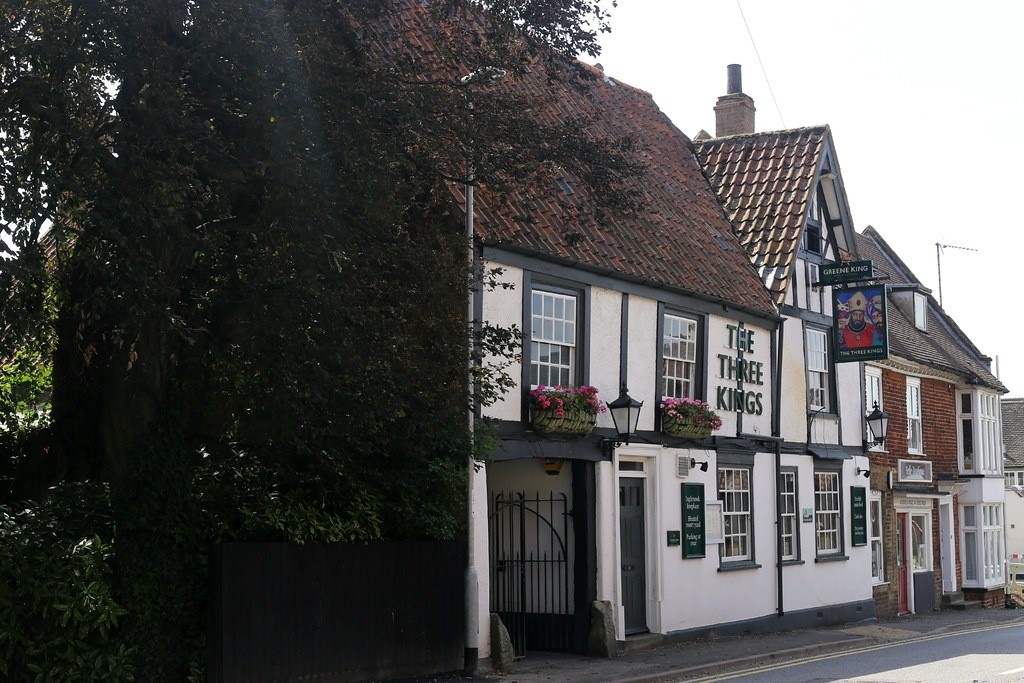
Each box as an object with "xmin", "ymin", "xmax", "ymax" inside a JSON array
[
  {"xmin": 847, "ymin": 292, "xmax": 867, "ymax": 313},
  {"xmin": 868, "ymin": 296, "xmax": 881, "ymax": 314},
  {"xmin": 838, "ymin": 304, "xmax": 849, "ymax": 319}
]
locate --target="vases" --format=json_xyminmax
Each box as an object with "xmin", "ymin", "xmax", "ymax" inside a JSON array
[
  {"xmin": 662, "ymin": 413, "xmax": 713, "ymax": 439},
  {"xmin": 532, "ymin": 404, "xmax": 598, "ymax": 436}
]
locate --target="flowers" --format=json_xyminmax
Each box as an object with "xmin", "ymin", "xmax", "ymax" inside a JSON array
[
  {"xmin": 531, "ymin": 384, "xmax": 606, "ymax": 415},
  {"xmin": 658, "ymin": 398, "xmax": 725, "ymax": 433}
]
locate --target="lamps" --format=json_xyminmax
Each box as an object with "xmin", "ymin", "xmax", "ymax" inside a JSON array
[
  {"xmin": 887, "ymin": 471, "xmax": 893, "ymax": 489},
  {"xmin": 857, "ymin": 467, "xmax": 872, "ymax": 478},
  {"xmin": 592, "ymin": 379, "xmax": 645, "ymax": 454},
  {"xmin": 690, "ymin": 457, "xmax": 709, "ymax": 473},
  {"xmin": 862, "ymin": 401, "xmax": 890, "ymax": 449}
]
